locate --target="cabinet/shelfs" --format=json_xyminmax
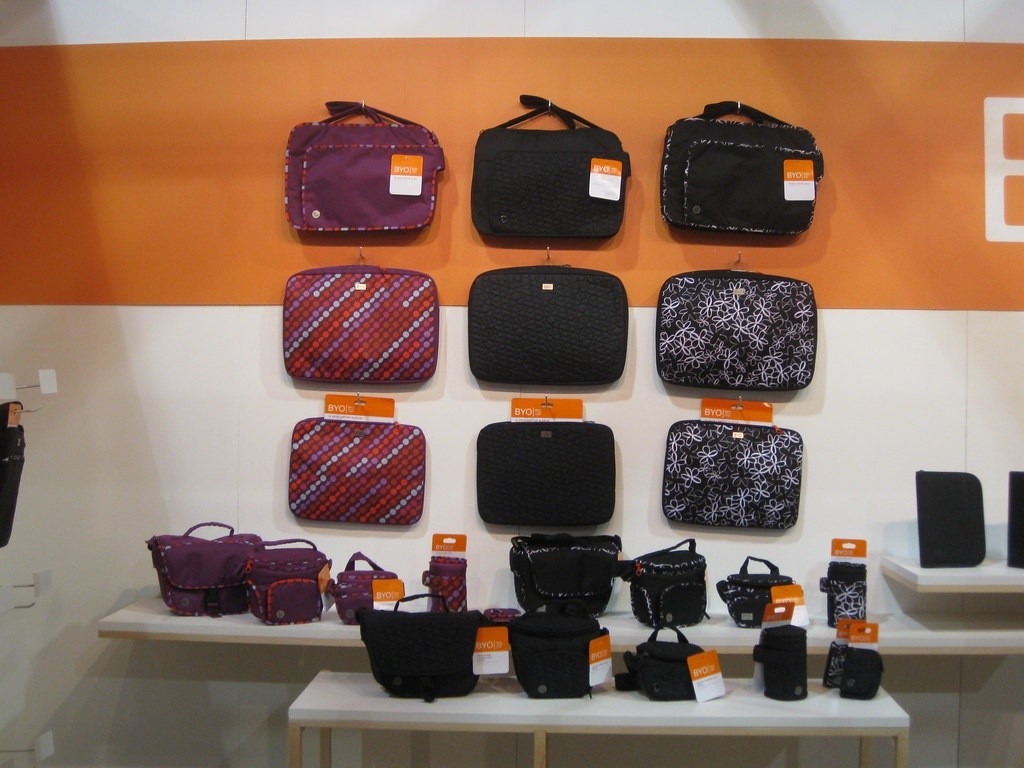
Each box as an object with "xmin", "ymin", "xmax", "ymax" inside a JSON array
[{"xmin": 97, "ymin": 559, "xmax": 1024, "ymax": 653}]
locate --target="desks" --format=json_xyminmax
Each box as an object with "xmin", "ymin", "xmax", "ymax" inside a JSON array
[{"xmin": 287, "ymin": 677, "xmax": 910, "ymax": 768}]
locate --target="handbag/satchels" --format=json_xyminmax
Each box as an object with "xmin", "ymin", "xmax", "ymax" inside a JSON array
[
  {"xmin": 145, "ymin": 521, "xmax": 266, "ymax": 619},
  {"xmin": 468, "ymin": 94, "xmax": 632, "ymax": 240},
  {"xmin": 717, "ymin": 556, "xmax": 796, "ymax": 628},
  {"xmin": 656, "ymin": 268, "xmax": 818, "ymax": 390},
  {"xmin": 466, "ymin": 264, "xmax": 630, "ymax": 386},
  {"xmin": 630, "ymin": 539, "xmax": 707, "ymax": 629},
  {"xmin": 661, "ymin": 418, "xmax": 804, "ymax": 531},
  {"xmin": 614, "ymin": 624, "xmax": 707, "ymax": 702},
  {"xmin": 331, "ymin": 552, "xmax": 401, "ymax": 626},
  {"xmin": 1, "ymin": 400, "xmax": 27, "ymax": 548},
  {"xmin": 282, "ymin": 262, "xmax": 440, "ymax": 385},
  {"xmin": 476, "ymin": 422, "xmax": 617, "ymax": 527},
  {"xmin": 243, "ymin": 539, "xmax": 332, "ymax": 626},
  {"xmin": 284, "ymin": 100, "xmax": 446, "ymax": 233},
  {"xmin": 288, "ymin": 417, "xmax": 427, "ymax": 526},
  {"xmin": 509, "ymin": 534, "xmax": 623, "ymax": 616},
  {"xmin": 657, "ymin": 101, "xmax": 824, "ymax": 239},
  {"xmin": 505, "ymin": 598, "xmax": 610, "ymax": 699},
  {"xmin": 752, "ymin": 624, "xmax": 809, "ymax": 701},
  {"xmin": 355, "ymin": 593, "xmax": 488, "ymax": 703}
]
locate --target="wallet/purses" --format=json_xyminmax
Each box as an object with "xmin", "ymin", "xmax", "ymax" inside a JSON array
[
  {"xmin": 822, "ymin": 641, "xmax": 884, "ymax": 701},
  {"xmin": 422, "ymin": 556, "xmax": 469, "ymax": 616},
  {"xmin": 1007, "ymin": 470, "xmax": 1024, "ymax": 570},
  {"xmin": 915, "ymin": 469, "xmax": 987, "ymax": 570},
  {"xmin": 819, "ymin": 561, "xmax": 869, "ymax": 634},
  {"xmin": 484, "ymin": 608, "xmax": 521, "ymax": 623}
]
